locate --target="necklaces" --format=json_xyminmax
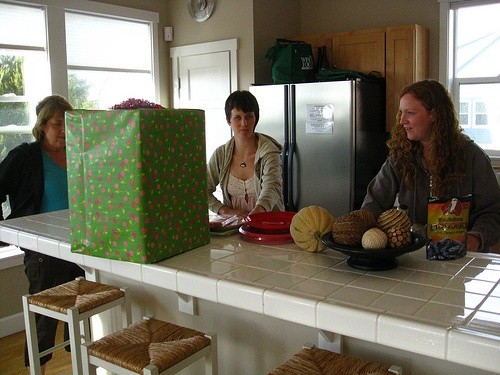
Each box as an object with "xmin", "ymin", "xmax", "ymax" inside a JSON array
[{"xmin": 235, "ymin": 142, "xmax": 251, "ymax": 168}]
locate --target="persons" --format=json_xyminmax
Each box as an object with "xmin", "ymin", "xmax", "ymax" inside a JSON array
[
  {"xmin": 207, "ymin": 90, "xmax": 285, "ymax": 219},
  {"xmin": 361, "ymin": 80, "xmax": 500, "ymax": 253},
  {"xmin": 0, "ymin": 94, "xmax": 91, "ymax": 375}
]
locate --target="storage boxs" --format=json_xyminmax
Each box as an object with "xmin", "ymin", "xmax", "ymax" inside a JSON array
[{"xmin": 64, "ymin": 98, "xmax": 212, "ymax": 266}]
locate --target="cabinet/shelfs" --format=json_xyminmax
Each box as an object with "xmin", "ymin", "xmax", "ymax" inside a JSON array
[{"xmin": 290, "ymin": 24, "xmax": 428, "ymax": 132}]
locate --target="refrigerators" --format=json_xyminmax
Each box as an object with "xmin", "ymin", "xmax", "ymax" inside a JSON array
[{"xmin": 248, "ymin": 78, "xmax": 387, "ymax": 219}]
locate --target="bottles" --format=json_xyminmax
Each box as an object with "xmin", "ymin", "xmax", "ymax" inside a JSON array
[{"xmin": 316, "ymin": 46, "xmax": 329, "ymax": 71}]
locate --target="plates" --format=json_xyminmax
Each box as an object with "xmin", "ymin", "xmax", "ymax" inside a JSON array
[{"xmin": 239, "ymin": 211, "xmax": 298, "ymax": 243}]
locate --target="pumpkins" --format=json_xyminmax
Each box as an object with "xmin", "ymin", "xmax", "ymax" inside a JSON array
[{"xmin": 290, "ymin": 205, "xmax": 337, "ymax": 253}]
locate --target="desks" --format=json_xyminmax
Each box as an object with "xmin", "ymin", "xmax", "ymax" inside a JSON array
[{"xmin": 0, "ymin": 212, "xmax": 500, "ymax": 370}]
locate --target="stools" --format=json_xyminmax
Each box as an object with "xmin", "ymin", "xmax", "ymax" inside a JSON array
[
  {"xmin": 268, "ymin": 342, "xmax": 402, "ymax": 375},
  {"xmin": 83, "ymin": 316, "xmax": 218, "ymax": 375},
  {"xmin": 23, "ymin": 277, "xmax": 130, "ymax": 375}
]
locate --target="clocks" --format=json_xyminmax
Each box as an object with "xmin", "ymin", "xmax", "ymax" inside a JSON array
[{"xmin": 187, "ymin": 0, "xmax": 214, "ymax": 23}]
